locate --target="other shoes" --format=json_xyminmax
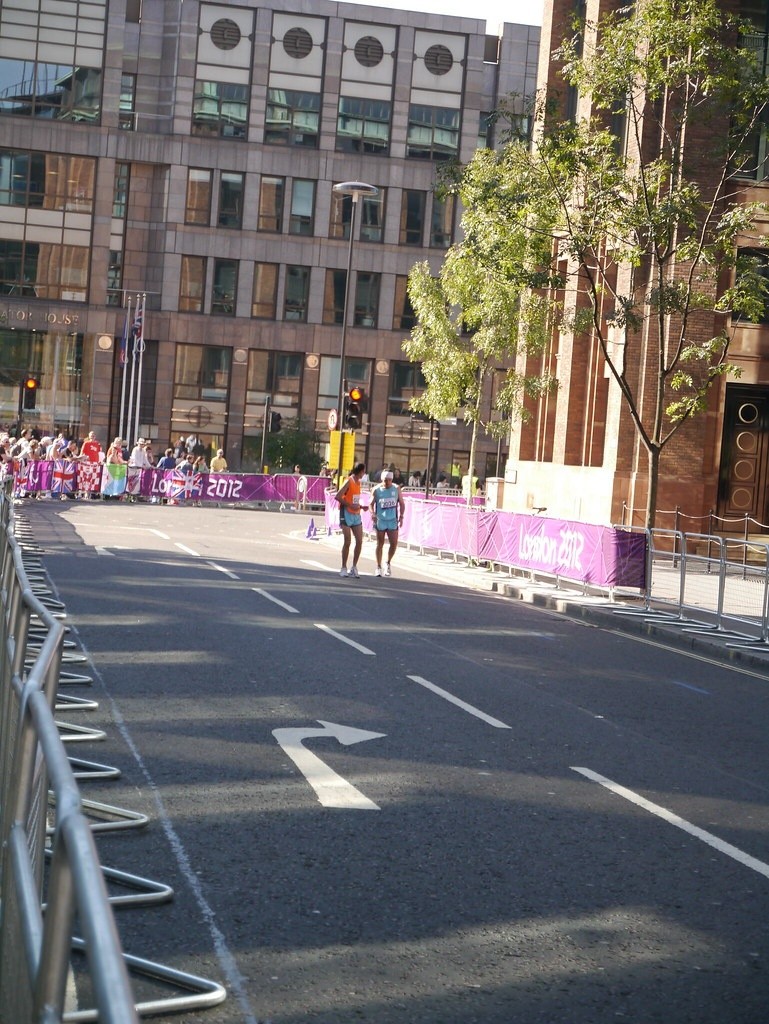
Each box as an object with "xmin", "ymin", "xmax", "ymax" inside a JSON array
[
  {"xmin": 384, "ymin": 562, "xmax": 391, "ymax": 576},
  {"xmin": 350, "ymin": 566, "xmax": 359, "ymax": 578},
  {"xmin": 339, "ymin": 567, "xmax": 349, "ymax": 577},
  {"xmin": 375, "ymin": 566, "xmax": 382, "ymax": 577}
]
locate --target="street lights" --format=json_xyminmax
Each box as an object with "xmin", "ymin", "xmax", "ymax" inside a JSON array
[{"xmin": 335, "ymin": 179, "xmax": 377, "ymax": 492}]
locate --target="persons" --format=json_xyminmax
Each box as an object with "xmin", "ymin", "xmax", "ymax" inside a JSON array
[
  {"xmin": 461, "ymin": 466, "xmax": 482, "ymax": 497},
  {"xmin": 0, "ymin": 423, "xmax": 457, "ymax": 511},
  {"xmin": 334, "ymin": 462, "xmax": 370, "ymax": 578},
  {"xmin": 368, "ymin": 469, "xmax": 405, "ymax": 577}
]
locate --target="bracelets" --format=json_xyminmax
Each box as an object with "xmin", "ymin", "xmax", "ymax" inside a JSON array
[
  {"xmin": 349, "ymin": 503, "xmax": 352, "ymax": 507},
  {"xmin": 400, "ymin": 516, "xmax": 404, "ymax": 519}
]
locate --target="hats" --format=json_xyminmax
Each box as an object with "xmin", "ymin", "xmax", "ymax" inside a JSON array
[{"xmin": 136, "ymin": 438, "xmax": 147, "ymax": 445}]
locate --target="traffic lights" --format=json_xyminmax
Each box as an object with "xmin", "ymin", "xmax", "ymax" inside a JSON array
[
  {"xmin": 268, "ymin": 411, "xmax": 282, "ymax": 433},
  {"xmin": 24, "ymin": 377, "xmax": 37, "ymax": 409},
  {"xmin": 346, "ymin": 386, "xmax": 364, "ymax": 429}
]
